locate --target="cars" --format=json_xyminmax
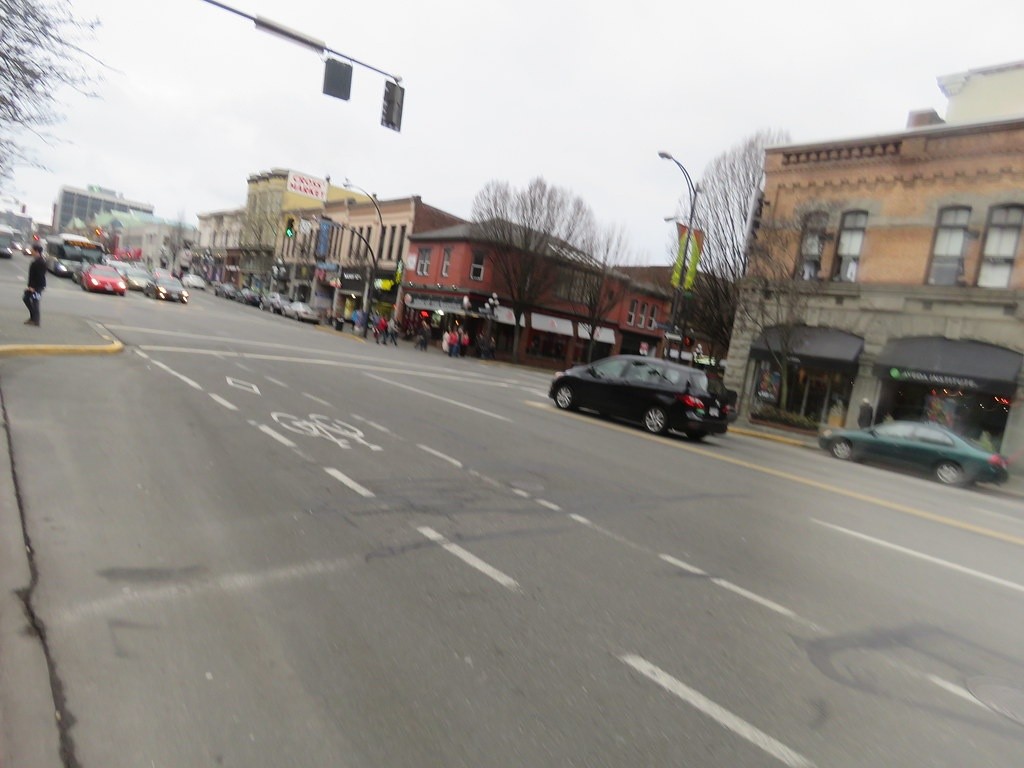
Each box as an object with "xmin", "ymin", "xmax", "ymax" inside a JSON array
[
  {"xmin": 214, "ymin": 282, "xmax": 240, "ymax": 300},
  {"xmin": 143, "ymin": 275, "xmax": 189, "ymax": 303},
  {"xmin": 151, "ymin": 268, "xmax": 171, "ymax": 279},
  {"xmin": 548, "ymin": 357, "xmax": 730, "ymax": 441},
  {"xmin": 235, "ymin": 289, "xmax": 262, "ymax": 305},
  {"xmin": 816, "ymin": 418, "xmax": 1008, "ymax": 488},
  {"xmin": 73, "ymin": 260, "xmax": 126, "ymax": 296},
  {"xmin": 106, "ymin": 260, "xmax": 150, "ymax": 290},
  {"xmin": 258, "ymin": 293, "xmax": 291, "ymax": 314},
  {"xmin": 724, "ymin": 389, "xmax": 737, "ymax": 422},
  {"xmin": 182, "ymin": 274, "xmax": 205, "ymax": 290},
  {"xmin": 282, "ymin": 301, "xmax": 321, "ymax": 324}
]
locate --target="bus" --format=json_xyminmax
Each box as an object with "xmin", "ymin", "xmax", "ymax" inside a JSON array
[
  {"xmin": 0, "ymin": 224, "xmax": 14, "ymax": 258},
  {"xmin": 23, "ymin": 231, "xmax": 41, "ymax": 254},
  {"xmin": 12, "ymin": 230, "xmax": 24, "ymax": 251},
  {"xmin": 42, "ymin": 235, "xmax": 105, "ymax": 276}
]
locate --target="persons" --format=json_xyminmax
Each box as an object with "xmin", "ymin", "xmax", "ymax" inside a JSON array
[
  {"xmin": 352, "ymin": 309, "xmax": 432, "ymax": 352},
  {"xmin": 442, "ymin": 327, "xmax": 468, "ymax": 358},
  {"xmin": 21, "ymin": 243, "xmax": 46, "ymax": 327}
]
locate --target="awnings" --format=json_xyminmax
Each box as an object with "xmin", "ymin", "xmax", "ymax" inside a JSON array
[
  {"xmin": 490, "ymin": 303, "xmax": 615, "ymax": 345},
  {"xmin": 746, "ymin": 325, "xmax": 865, "ymax": 375},
  {"xmin": 870, "ymin": 337, "xmax": 1023, "ymax": 396}
]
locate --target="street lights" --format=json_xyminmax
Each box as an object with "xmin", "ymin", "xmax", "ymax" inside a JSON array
[
  {"xmin": 658, "ymin": 151, "xmax": 697, "ymax": 356},
  {"xmin": 343, "ymin": 182, "xmax": 384, "ymax": 337}
]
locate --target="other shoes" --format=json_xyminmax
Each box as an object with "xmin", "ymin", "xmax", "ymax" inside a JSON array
[{"xmin": 25, "ymin": 320, "xmax": 38, "ymax": 325}]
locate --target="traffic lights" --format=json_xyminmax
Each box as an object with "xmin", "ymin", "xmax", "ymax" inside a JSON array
[
  {"xmin": 286, "ymin": 215, "xmax": 297, "ymax": 238},
  {"xmin": 683, "ymin": 337, "xmax": 694, "ymax": 347}
]
